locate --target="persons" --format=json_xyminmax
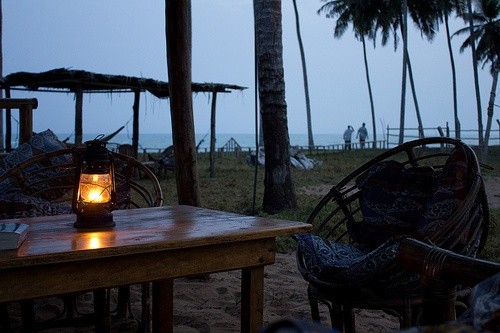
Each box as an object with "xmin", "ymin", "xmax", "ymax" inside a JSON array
[
  {"xmin": 355, "ymin": 122, "xmax": 369, "ymax": 148},
  {"xmin": 343, "ymin": 125, "xmax": 355, "ymax": 150}
]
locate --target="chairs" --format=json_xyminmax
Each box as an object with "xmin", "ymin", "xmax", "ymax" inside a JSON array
[
  {"xmin": 0, "ymin": 145, "xmax": 164, "ymax": 332},
  {"xmin": 296, "ymin": 136, "xmax": 490, "ymax": 332},
  {"xmin": 157, "ymin": 144, "xmax": 176, "ymax": 178}
]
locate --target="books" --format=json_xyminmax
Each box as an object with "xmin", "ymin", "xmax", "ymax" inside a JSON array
[{"xmin": 0, "ymin": 223, "xmax": 30, "ymax": 249}]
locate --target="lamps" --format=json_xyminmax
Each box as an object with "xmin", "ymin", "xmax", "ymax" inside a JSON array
[{"xmin": 69, "ymin": 139, "xmax": 118, "ymax": 229}]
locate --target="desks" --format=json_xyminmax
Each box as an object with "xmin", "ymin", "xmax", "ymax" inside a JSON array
[
  {"xmin": 0, "ymin": 203, "xmax": 315, "ymax": 332},
  {"xmin": 136, "ymin": 160, "xmax": 159, "ymax": 179}
]
